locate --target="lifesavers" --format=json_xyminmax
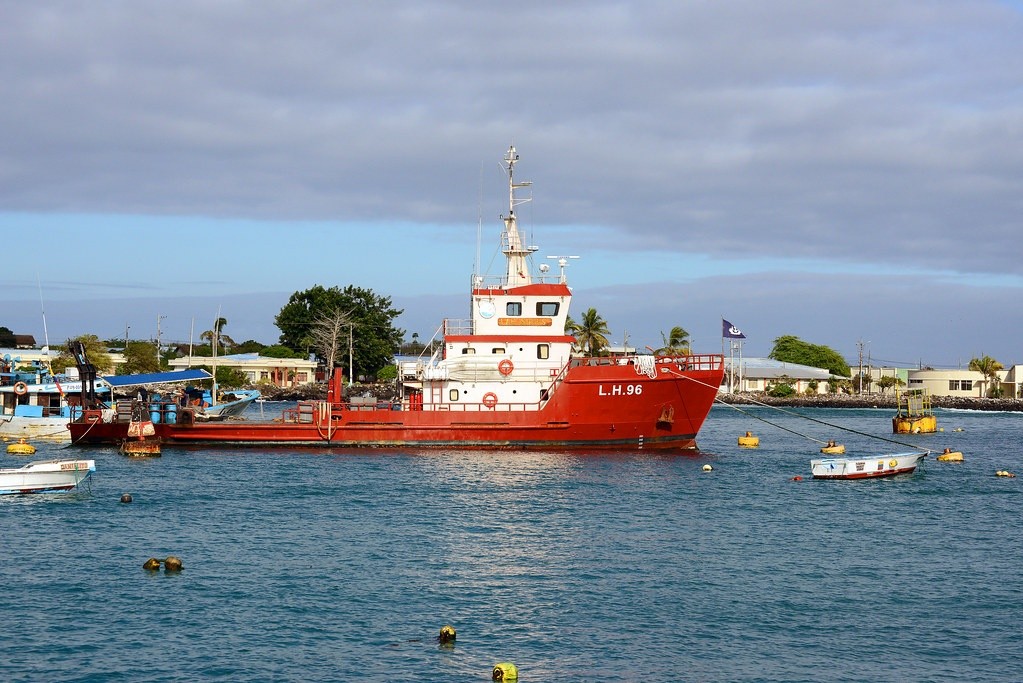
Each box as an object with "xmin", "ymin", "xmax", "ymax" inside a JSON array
[
  {"xmin": 14, "ymin": 381, "xmax": 27, "ymax": 396},
  {"xmin": 499, "ymin": 359, "xmax": 514, "ymax": 374},
  {"xmin": 482, "ymin": 392, "xmax": 498, "ymax": 407}
]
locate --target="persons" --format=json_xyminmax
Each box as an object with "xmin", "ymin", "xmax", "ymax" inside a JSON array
[{"xmin": 174, "ymin": 389, "xmax": 188, "ymax": 407}]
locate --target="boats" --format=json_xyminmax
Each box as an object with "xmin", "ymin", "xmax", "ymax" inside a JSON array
[
  {"xmin": 0, "ymin": 458, "xmax": 95, "ymax": 499},
  {"xmin": 66, "ymin": 136, "xmax": 726, "ymax": 455},
  {"xmin": 810, "ymin": 449, "xmax": 927, "ymax": 479}
]
